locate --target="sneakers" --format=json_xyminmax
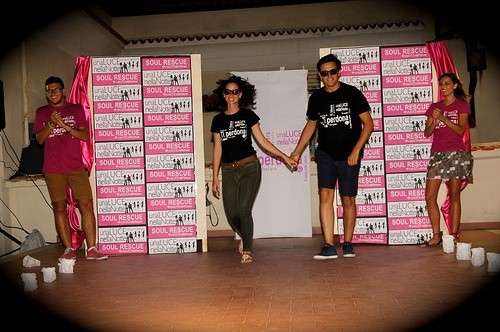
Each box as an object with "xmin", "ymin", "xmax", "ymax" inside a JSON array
[
  {"xmin": 56, "ymin": 248, "xmax": 77, "ymax": 262},
  {"xmin": 342, "ymin": 242, "xmax": 357, "ymax": 258},
  {"xmin": 314, "ymin": 243, "xmax": 338, "ymax": 260},
  {"xmin": 87, "ymin": 247, "xmax": 109, "ymax": 260}
]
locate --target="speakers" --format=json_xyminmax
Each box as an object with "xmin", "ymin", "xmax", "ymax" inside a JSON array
[{"xmin": 0, "ymin": 80, "xmax": 5, "ymax": 132}]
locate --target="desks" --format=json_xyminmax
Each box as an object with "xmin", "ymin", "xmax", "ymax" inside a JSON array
[
  {"xmin": 459, "ymin": 141, "xmax": 500, "ymax": 230},
  {"xmin": 5, "ymin": 174, "xmax": 58, "ymax": 253}
]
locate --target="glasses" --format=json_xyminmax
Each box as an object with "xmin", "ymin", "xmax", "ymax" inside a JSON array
[
  {"xmin": 46, "ymin": 88, "xmax": 63, "ymax": 94},
  {"xmin": 223, "ymin": 88, "xmax": 241, "ymax": 95},
  {"xmin": 320, "ymin": 68, "xmax": 338, "ymax": 77}
]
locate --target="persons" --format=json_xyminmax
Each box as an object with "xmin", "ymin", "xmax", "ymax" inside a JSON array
[
  {"xmin": 210, "ymin": 73, "xmax": 297, "ymax": 263},
  {"xmin": 419, "ymin": 72, "xmax": 472, "ymax": 247},
  {"xmin": 31, "ymin": 76, "xmax": 108, "ymax": 260},
  {"xmin": 290, "ymin": 54, "xmax": 374, "ymax": 259}
]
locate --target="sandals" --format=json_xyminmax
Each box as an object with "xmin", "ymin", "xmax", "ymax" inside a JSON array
[
  {"xmin": 419, "ymin": 231, "xmax": 443, "ymax": 247},
  {"xmin": 450, "ymin": 233, "xmax": 461, "ymax": 247}
]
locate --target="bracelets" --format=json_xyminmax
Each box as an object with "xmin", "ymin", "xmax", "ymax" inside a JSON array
[
  {"xmin": 441, "ymin": 116, "xmax": 447, "ymax": 122},
  {"xmin": 48, "ymin": 121, "xmax": 55, "ymax": 128},
  {"xmin": 67, "ymin": 128, "xmax": 72, "ymax": 132}
]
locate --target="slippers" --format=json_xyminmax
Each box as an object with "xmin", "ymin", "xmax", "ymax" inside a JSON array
[
  {"xmin": 239, "ymin": 239, "xmax": 244, "ymax": 254},
  {"xmin": 240, "ymin": 255, "xmax": 252, "ymax": 264}
]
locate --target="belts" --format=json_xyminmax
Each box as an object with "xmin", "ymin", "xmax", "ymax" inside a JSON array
[{"xmin": 222, "ymin": 154, "xmax": 257, "ymax": 167}]
